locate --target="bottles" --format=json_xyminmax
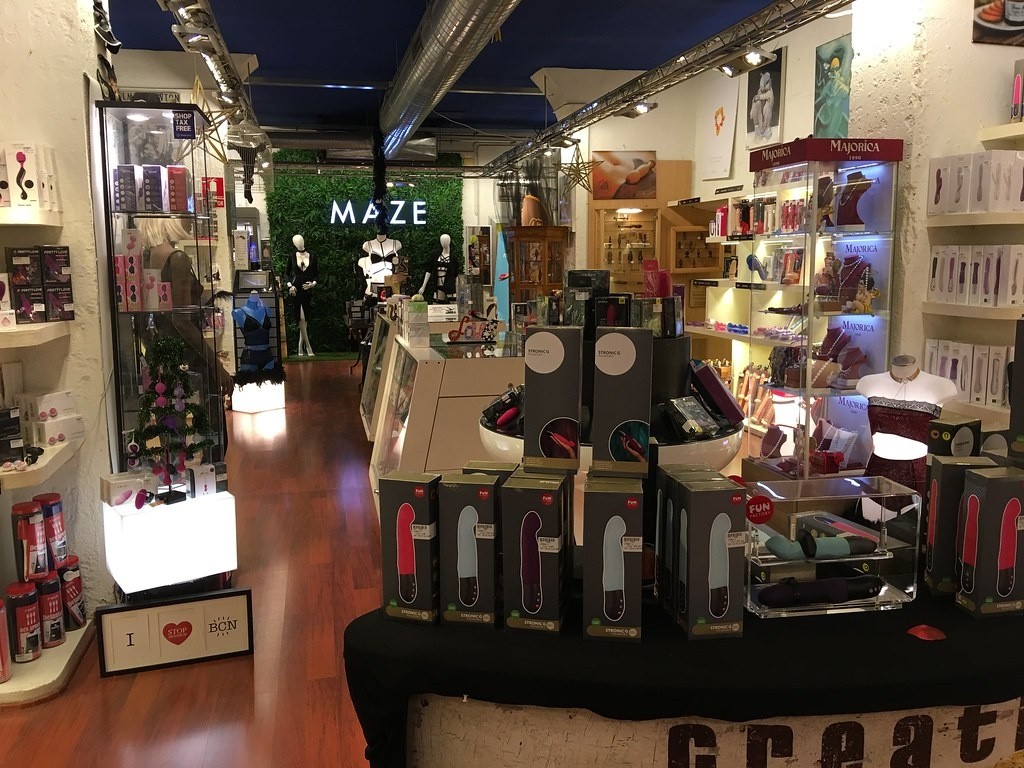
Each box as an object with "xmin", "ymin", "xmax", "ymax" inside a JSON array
[{"xmin": 0, "ymin": 492, "xmax": 87, "ymax": 684}]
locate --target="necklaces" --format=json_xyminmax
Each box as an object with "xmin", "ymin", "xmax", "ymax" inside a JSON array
[
  {"xmin": 820, "ymin": 330, "xmax": 845, "ymax": 356},
  {"xmin": 812, "ymin": 358, "xmax": 833, "ymax": 386},
  {"xmin": 838, "ymin": 256, "xmax": 864, "ymax": 296},
  {"xmin": 819, "ymin": 179, "xmax": 833, "ymax": 197},
  {"xmin": 760, "ymin": 430, "xmax": 783, "ymax": 459},
  {"xmin": 840, "ymin": 175, "xmax": 865, "ymax": 206},
  {"xmin": 840, "ymin": 353, "xmax": 863, "ymax": 372},
  {"xmin": 816, "ymin": 422, "xmax": 855, "ymax": 454}
]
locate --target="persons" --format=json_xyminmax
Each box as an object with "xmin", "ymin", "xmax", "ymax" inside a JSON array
[
  {"xmin": 419, "ymin": 234, "xmax": 458, "ymax": 305},
  {"xmin": 232, "ymin": 290, "xmax": 274, "ymax": 371},
  {"xmin": 285, "ymin": 235, "xmax": 318, "ymax": 357},
  {"xmin": 794, "ymin": 429, "xmax": 805, "ymax": 460},
  {"xmin": 849, "ymin": 277, "xmax": 875, "ymax": 313},
  {"xmin": 856, "ymin": 355, "xmax": 958, "ymax": 521},
  {"xmin": 135, "ymin": 214, "xmax": 234, "ymax": 466},
  {"xmin": 362, "ymin": 228, "xmax": 402, "ymax": 282}
]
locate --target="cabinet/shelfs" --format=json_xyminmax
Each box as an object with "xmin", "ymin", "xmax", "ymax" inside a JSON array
[
  {"xmin": 919, "ymin": 119, "xmax": 1023, "ymax": 424},
  {"xmin": 744, "ymin": 136, "xmax": 903, "ymax": 484},
  {"xmin": 508, "ymin": 224, "xmax": 574, "ymax": 322},
  {"xmin": 95, "ymin": 102, "xmax": 230, "ymax": 480},
  {"xmin": 0, "ymin": 206, "xmax": 93, "ymax": 710}
]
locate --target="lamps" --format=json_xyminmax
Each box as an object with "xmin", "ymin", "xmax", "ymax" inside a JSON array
[
  {"xmin": 719, "ymin": 45, "xmax": 778, "ymax": 78},
  {"xmin": 613, "ymin": 100, "xmax": 659, "ymax": 120}
]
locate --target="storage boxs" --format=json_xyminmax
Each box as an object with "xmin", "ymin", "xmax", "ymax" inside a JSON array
[{"xmin": 0, "ymin": 142, "xmax": 1023, "ymax": 640}]
